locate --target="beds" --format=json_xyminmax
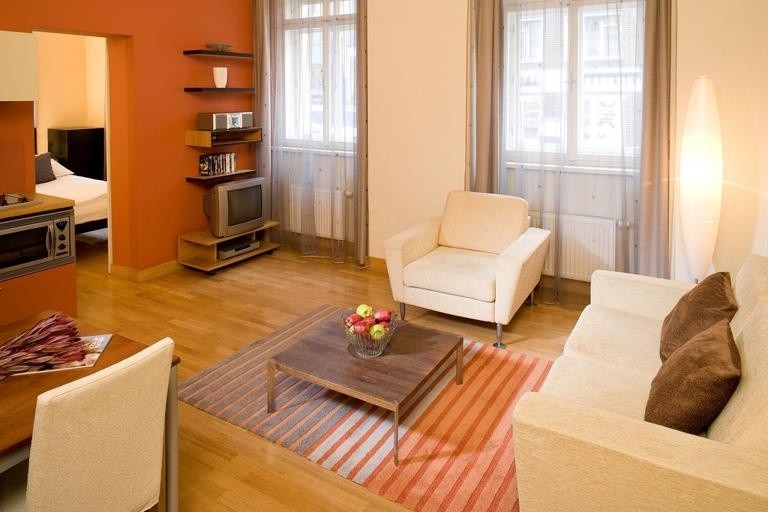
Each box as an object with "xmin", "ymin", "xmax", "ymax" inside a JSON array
[{"xmin": 34, "ymin": 125, "xmax": 107, "ymax": 237}]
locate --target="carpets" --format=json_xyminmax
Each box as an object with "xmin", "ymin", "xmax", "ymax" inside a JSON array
[{"xmin": 177, "ymin": 304, "xmax": 554, "ymax": 512}]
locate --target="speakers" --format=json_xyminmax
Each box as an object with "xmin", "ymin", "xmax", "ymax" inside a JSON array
[{"xmin": 197, "ymin": 112, "xmax": 253, "ymax": 130}]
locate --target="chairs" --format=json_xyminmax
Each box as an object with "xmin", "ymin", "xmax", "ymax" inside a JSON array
[
  {"xmin": 381, "ymin": 186, "xmax": 549, "ymax": 352},
  {"xmin": 0, "ymin": 335, "xmax": 174, "ymax": 512}
]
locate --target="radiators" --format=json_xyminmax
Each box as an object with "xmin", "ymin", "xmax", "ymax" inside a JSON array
[
  {"xmin": 526, "ymin": 209, "xmax": 617, "ymax": 284},
  {"xmin": 286, "ymin": 182, "xmax": 354, "ymax": 244}
]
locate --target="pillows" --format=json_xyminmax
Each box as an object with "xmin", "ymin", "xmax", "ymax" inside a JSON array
[
  {"xmin": 645, "ymin": 319, "xmax": 741, "ymax": 434},
  {"xmin": 34, "ymin": 150, "xmax": 54, "ymax": 184},
  {"xmin": 49, "ymin": 158, "xmax": 73, "ymax": 180},
  {"xmin": 661, "ymin": 271, "xmax": 736, "ymax": 362}
]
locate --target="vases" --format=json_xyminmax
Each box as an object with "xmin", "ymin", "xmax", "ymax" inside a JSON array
[{"xmin": 213, "ymin": 66, "xmax": 228, "ymax": 89}]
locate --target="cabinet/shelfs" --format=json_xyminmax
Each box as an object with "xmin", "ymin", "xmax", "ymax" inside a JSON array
[
  {"xmin": 176, "ymin": 49, "xmax": 282, "ymax": 274},
  {"xmin": 47, "ymin": 127, "xmax": 106, "ymax": 181}
]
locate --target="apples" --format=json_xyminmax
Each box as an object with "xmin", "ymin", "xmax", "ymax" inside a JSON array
[{"xmin": 344, "ymin": 304, "xmax": 392, "ymax": 339}]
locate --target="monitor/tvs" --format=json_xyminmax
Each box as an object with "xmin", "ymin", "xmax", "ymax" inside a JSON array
[{"xmin": 202, "ymin": 177, "xmax": 267, "ymax": 239}]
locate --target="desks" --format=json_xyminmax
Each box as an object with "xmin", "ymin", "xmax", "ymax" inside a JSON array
[{"xmin": 0, "ymin": 308, "xmax": 182, "ymax": 512}]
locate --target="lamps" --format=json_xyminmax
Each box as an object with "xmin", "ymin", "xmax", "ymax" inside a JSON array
[{"xmin": 674, "ymin": 74, "xmax": 721, "ymax": 287}]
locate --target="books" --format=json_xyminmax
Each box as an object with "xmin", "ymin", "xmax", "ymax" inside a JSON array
[
  {"xmin": 0, "ymin": 334, "xmax": 113, "ymax": 376},
  {"xmin": 199, "ymin": 153, "xmax": 237, "ymax": 175}
]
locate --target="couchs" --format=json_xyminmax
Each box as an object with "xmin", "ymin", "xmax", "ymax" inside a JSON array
[{"xmin": 509, "ymin": 252, "xmax": 768, "ymax": 512}]
